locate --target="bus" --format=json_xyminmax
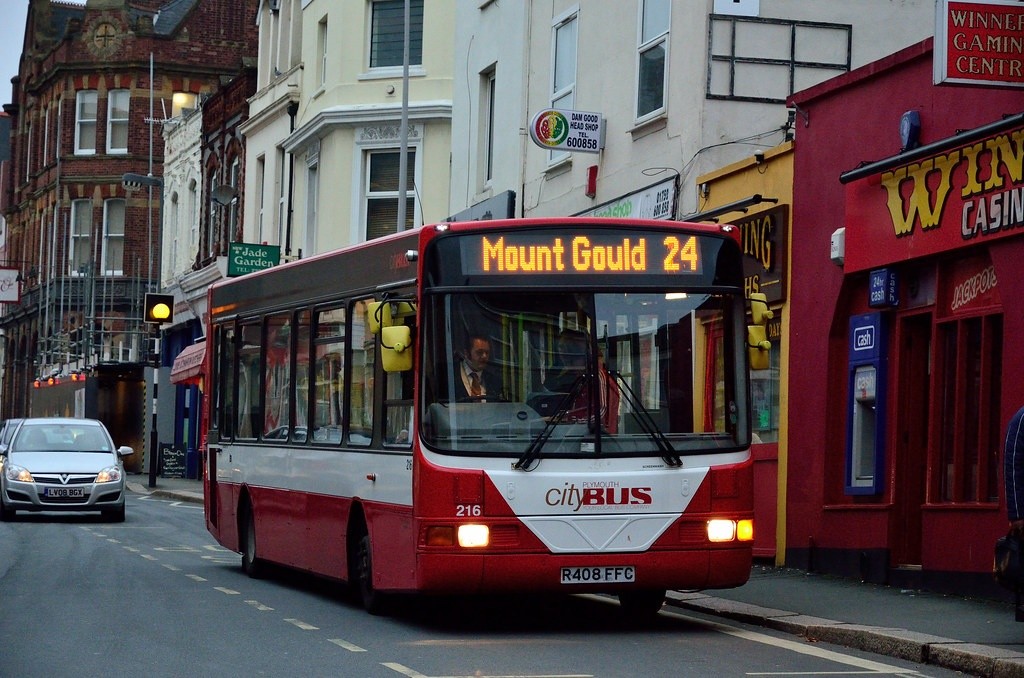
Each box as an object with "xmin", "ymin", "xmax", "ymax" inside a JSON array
[{"xmin": 195, "ymin": 215, "xmax": 774, "ymax": 634}]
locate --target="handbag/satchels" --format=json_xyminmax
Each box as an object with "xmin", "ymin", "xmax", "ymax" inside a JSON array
[{"xmin": 994, "ymin": 528, "xmax": 1024, "ymax": 589}]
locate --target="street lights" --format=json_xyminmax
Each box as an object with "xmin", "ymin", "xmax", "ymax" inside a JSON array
[{"xmin": 142, "ymin": 303, "xmax": 170, "ymax": 488}]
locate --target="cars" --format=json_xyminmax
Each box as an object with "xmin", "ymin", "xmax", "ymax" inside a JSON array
[
  {"xmin": 0, "ymin": 418, "xmax": 134, "ymax": 522},
  {"xmin": 0, "ymin": 418, "xmax": 77, "ymax": 455}
]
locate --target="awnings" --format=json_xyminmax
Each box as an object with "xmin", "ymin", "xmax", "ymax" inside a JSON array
[{"xmin": 170, "ymin": 341, "xmax": 206, "ymax": 385}]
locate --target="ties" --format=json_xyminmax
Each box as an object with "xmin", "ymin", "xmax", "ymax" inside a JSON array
[{"xmin": 470, "ymin": 373, "xmax": 481, "ymax": 402}]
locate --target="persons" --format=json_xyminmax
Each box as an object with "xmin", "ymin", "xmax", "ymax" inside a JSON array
[
  {"xmin": 1004, "ymin": 406, "xmax": 1024, "ymax": 622},
  {"xmin": 434, "ymin": 334, "xmax": 505, "ymax": 407},
  {"xmin": 540, "ymin": 330, "xmax": 619, "ymax": 434}
]
